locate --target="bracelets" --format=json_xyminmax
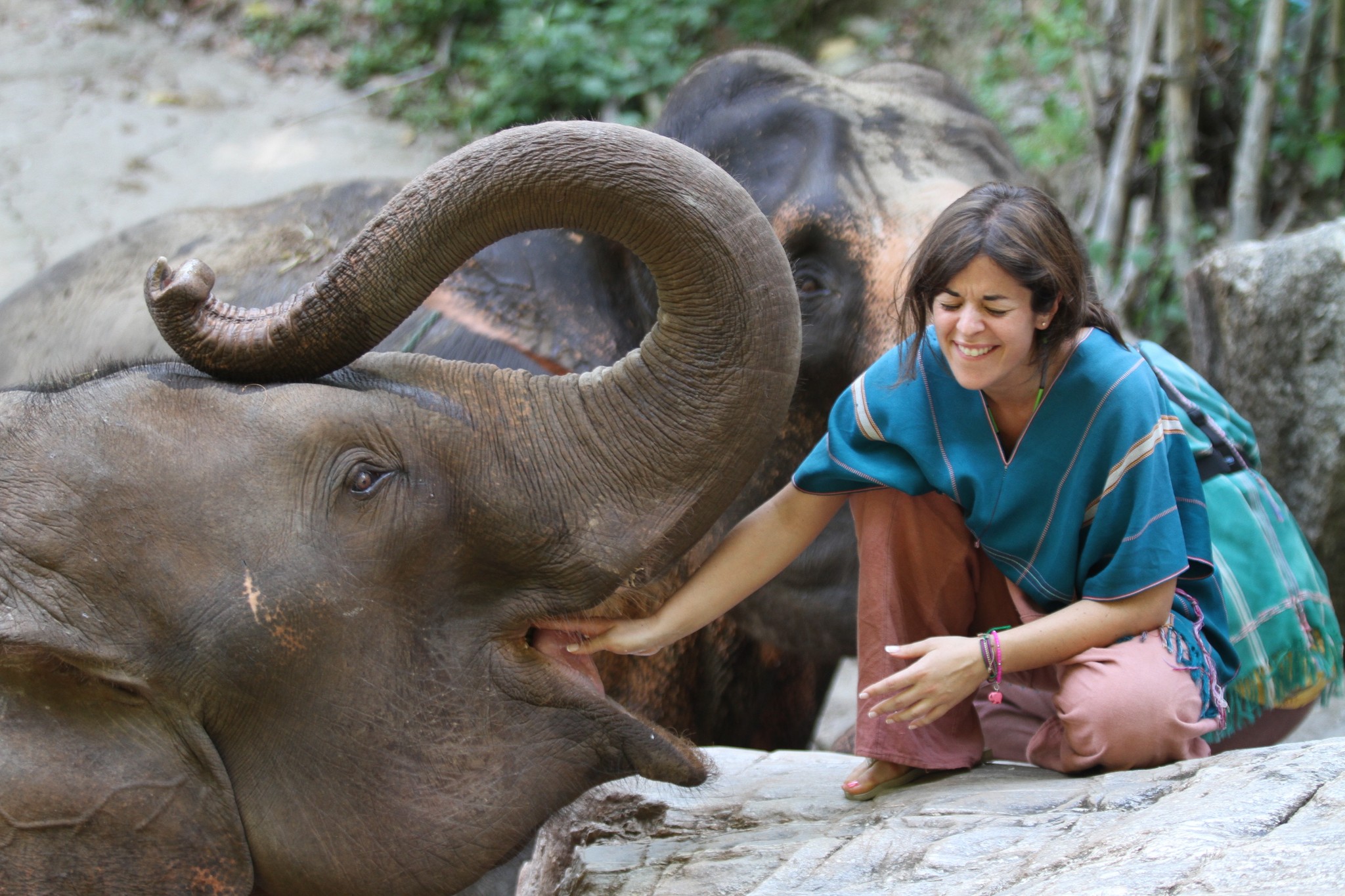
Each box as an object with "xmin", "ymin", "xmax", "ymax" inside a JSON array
[{"xmin": 975, "ymin": 625, "xmax": 1011, "ymax": 705}]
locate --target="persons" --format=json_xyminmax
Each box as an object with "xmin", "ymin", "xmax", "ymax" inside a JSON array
[
  {"xmin": 1129, "ymin": 327, "xmax": 1343, "ymax": 755},
  {"xmin": 529, "ymin": 179, "xmax": 1232, "ymax": 804}
]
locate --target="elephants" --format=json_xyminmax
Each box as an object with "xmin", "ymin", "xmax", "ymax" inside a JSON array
[{"xmin": 0, "ymin": 47, "xmax": 1102, "ymax": 896}]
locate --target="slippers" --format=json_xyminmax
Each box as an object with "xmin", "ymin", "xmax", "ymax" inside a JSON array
[{"xmin": 842, "ymin": 755, "xmax": 928, "ymax": 800}]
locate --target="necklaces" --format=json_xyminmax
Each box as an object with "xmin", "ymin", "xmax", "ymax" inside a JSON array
[{"xmin": 984, "ymin": 349, "xmax": 1046, "ymax": 456}]
